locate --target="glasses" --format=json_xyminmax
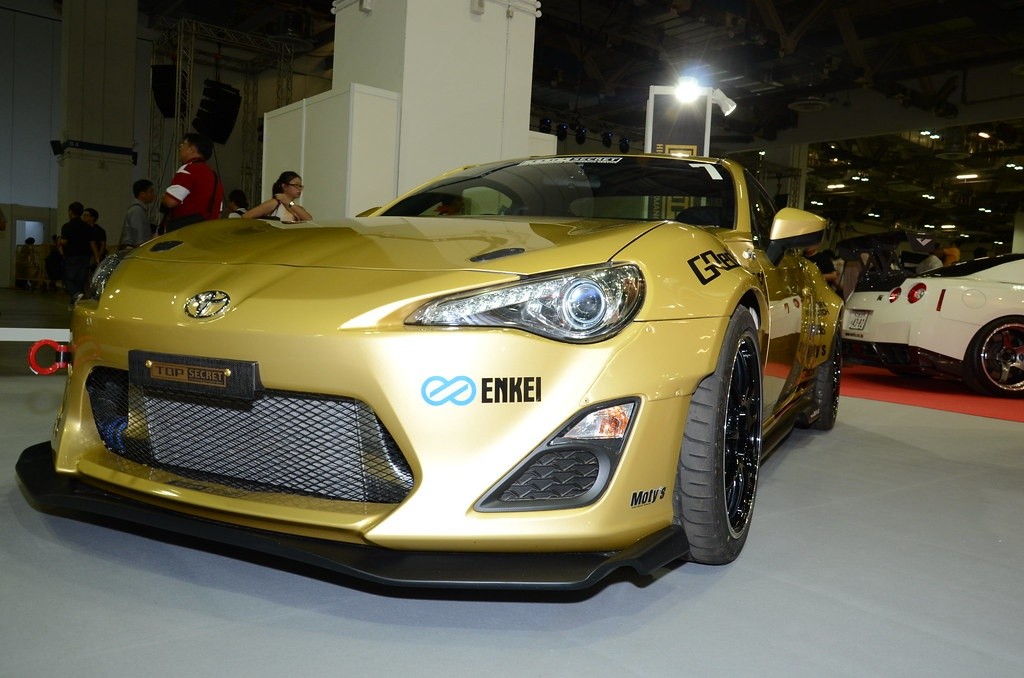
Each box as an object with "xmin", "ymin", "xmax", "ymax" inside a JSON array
[
  {"xmin": 180, "ymin": 142, "xmax": 189, "ymax": 148},
  {"xmin": 282, "ymin": 181, "xmax": 305, "ymax": 191}
]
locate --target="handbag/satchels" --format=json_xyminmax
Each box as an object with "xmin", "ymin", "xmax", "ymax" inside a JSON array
[{"xmin": 259, "ymin": 197, "xmax": 280, "ymax": 222}]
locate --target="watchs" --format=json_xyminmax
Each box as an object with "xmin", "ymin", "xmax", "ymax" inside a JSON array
[{"xmin": 289, "ymin": 201, "xmax": 294, "ymax": 207}]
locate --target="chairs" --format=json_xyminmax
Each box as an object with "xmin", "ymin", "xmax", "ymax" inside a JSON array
[{"xmin": 674, "ymin": 206, "xmax": 724, "ymax": 225}]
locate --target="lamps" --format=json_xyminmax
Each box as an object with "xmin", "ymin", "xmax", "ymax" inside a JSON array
[
  {"xmin": 575, "ymin": 127, "xmax": 587, "ymax": 145},
  {"xmin": 618, "ymin": 138, "xmax": 630, "ymax": 154},
  {"xmin": 539, "ymin": 118, "xmax": 552, "ymax": 134},
  {"xmin": 556, "ymin": 123, "xmax": 569, "ymax": 141},
  {"xmin": 601, "ymin": 132, "xmax": 613, "ymax": 148},
  {"xmin": 712, "ymin": 88, "xmax": 737, "ymax": 117}
]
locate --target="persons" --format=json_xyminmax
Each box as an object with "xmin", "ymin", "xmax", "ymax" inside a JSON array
[
  {"xmin": 804, "ymin": 246, "xmax": 843, "ymax": 298},
  {"xmin": 228, "ymin": 189, "xmax": 249, "ymax": 218},
  {"xmin": 915, "ymin": 243, "xmax": 944, "ymax": 275},
  {"xmin": 942, "ymin": 240, "xmax": 962, "ymax": 266},
  {"xmin": 19, "ymin": 238, "xmax": 39, "ymax": 277},
  {"xmin": 119, "ymin": 179, "xmax": 155, "ymax": 246},
  {"xmin": 242, "ymin": 171, "xmax": 313, "ymax": 221},
  {"xmin": 161, "ymin": 132, "xmax": 224, "ymax": 233},
  {"xmin": 854, "ymin": 242, "xmax": 887, "ymax": 273},
  {"xmin": 60, "ymin": 201, "xmax": 100, "ymax": 311},
  {"xmin": 82, "ymin": 208, "xmax": 108, "ymax": 273}
]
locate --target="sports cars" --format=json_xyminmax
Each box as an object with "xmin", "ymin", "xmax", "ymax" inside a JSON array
[
  {"xmin": 49, "ymin": 148, "xmax": 848, "ymax": 597},
  {"xmin": 840, "ymin": 254, "xmax": 1023, "ymax": 398}
]
